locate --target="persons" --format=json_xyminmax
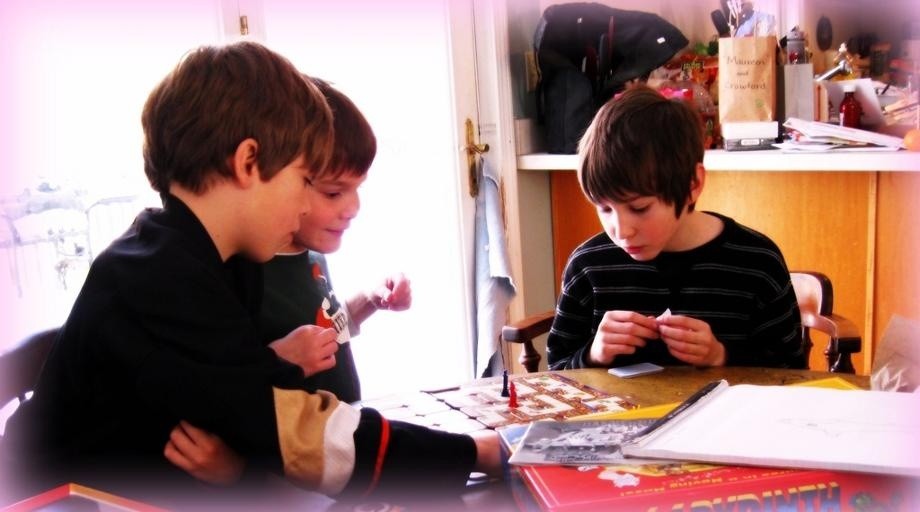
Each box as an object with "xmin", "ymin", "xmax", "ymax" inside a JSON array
[
  {"xmin": 546, "ymin": 82, "xmax": 809, "ymax": 370},
  {"xmin": 31, "ymin": 41, "xmax": 506, "ymax": 504},
  {"xmin": 264, "ymin": 78, "xmax": 412, "ymax": 404}
]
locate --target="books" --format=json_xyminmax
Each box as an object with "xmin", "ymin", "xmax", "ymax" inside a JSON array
[{"xmin": 494, "ymin": 377, "xmax": 920, "ymax": 512}]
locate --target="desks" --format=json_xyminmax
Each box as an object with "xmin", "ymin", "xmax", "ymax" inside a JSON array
[
  {"xmin": 1, "ymin": 369, "xmax": 918, "ymax": 512},
  {"xmin": 516, "ymin": 127, "xmax": 920, "ymax": 374}
]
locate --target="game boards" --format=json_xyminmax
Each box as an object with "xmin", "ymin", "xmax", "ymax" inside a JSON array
[{"xmin": 361, "ymin": 371, "xmax": 641, "ymax": 438}]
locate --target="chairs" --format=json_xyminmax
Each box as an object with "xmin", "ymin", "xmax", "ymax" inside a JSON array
[
  {"xmin": 0, "ymin": 327, "xmax": 66, "ymax": 512},
  {"xmin": 502, "ymin": 270, "xmax": 862, "ymax": 374}
]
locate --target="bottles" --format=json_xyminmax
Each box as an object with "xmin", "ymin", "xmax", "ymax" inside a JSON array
[
  {"xmin": 786, "ymin": 31, "xmax": 806, "ymax": 63},
  {"xmin": 837, "ymin": 84, "xmax": 862, "ymax": 129}
]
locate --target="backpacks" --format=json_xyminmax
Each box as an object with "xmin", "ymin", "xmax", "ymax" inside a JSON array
[{"xmin": 533, "ymin": 3, "xmax": 688, "ymax": 154}]
